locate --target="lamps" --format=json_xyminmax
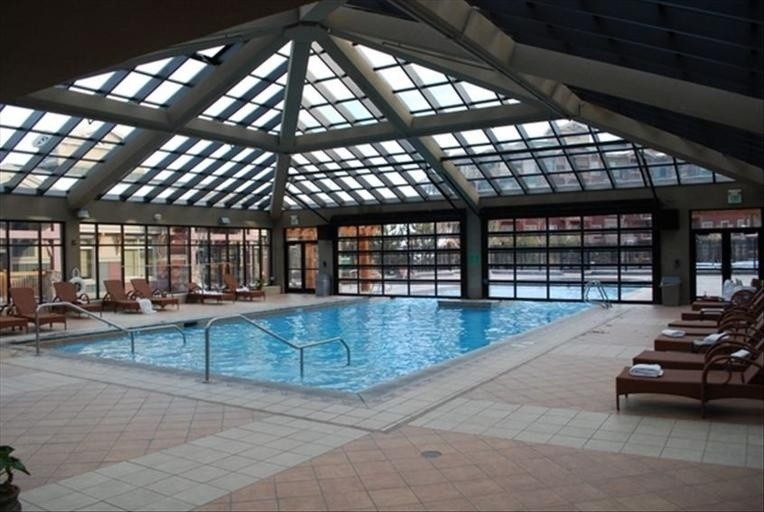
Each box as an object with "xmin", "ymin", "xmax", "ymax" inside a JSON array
[
  {"xmin": 217, "ymin": 216, "xmax": 230, "ymax": 225},
  {"xmin": 77, "ymin": 208, "xmax": 91, "ymax": 220}
]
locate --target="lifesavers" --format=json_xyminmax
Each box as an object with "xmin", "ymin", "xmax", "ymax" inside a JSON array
[
  {"xmin": 72, "ymin": 268, "xmax": 81, "ymax": 278},
  {"xmin": 70, "ymin": 277, "xmax": 86, "ymax": 297}
]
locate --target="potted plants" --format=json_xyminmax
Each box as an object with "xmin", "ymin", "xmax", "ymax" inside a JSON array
[{"xmin": 0, "ymin": 446, "xmax": 31, "ymax": 512}]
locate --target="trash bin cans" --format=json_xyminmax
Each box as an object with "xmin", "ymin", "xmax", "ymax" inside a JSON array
[
  {"xmin": 315, "ymin": 273, "xmax": 330, "ymax": 297},
  {"xmin": 659, "ymin": 276, "xmax": 682, "ymax": 306}
]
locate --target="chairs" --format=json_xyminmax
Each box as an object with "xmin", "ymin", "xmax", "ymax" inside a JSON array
[
  {"xmin": 615, "ymin": 278, "xmax": 764, "ymax": 419},
  {"xmin": 0, "ymin": 273, "xmax": 265, "ymax": 334}
]
